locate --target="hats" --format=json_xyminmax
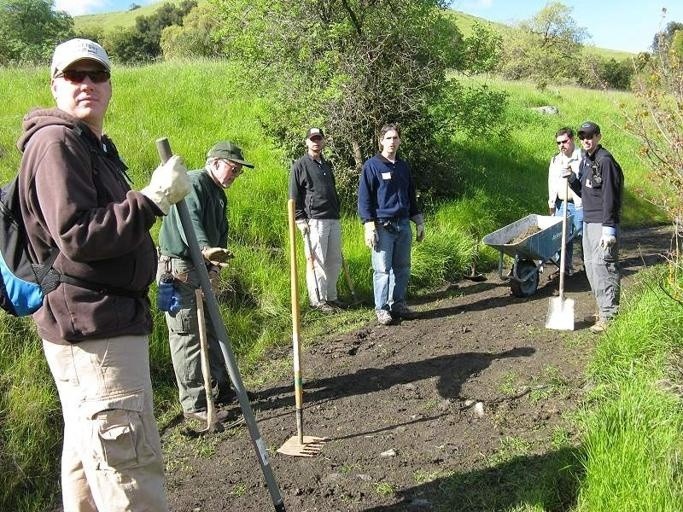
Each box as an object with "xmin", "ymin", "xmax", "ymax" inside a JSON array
[
  {"xmin": 51, "ymin": 38, "xmax": 111, "ymax": 85},
  {"xmin": 207, "ymin": 143, "xmax": 254, "ymax": 167},
  {"xmin": 308, "ymin": 128, "xmax": 324, "ymax": 139},
  {"xmin": 578, "ymin": 122, "xmax": 599, "ymax": 135}
]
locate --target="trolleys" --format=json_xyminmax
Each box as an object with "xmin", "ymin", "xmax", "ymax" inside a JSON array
[{"xmin": 481, "ymin": 212, "xmax": 582, "ymax": 299}]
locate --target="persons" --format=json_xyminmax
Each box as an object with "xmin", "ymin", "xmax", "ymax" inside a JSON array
[
  {"xmin": 562, "ymin": 121, "xmax": 625, "ymax": 332},
  {"xmin": 547, "ymin": 127, "xmax": 584, "ymax": 283},
  {"xmin": 359, "ymin": 124, "xmax": 424, "ymax": 325},
  {"xmin": 15, "ymin": 37, "xmax": 192, "ymax": 512},
  {"xmin": 287, "ymin": 128, "xmax": 349, "ymax": 312},
  {"xmin": 155, "ymin": 142, "xmax": 256, "ymax": 425}
]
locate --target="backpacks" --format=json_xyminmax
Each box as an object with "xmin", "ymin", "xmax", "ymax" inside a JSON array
[{"xmin": 0, "ymin": 173, "xmax": 60, "ymax": 317}]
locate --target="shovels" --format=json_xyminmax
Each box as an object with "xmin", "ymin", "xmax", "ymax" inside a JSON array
[
  {"xmin": 545, "ymin": 159, "xmax": 575, "ymax": 330},
  {"xmin": 463, "ymin": 235, "xmax": 488, "ymax": 282}
]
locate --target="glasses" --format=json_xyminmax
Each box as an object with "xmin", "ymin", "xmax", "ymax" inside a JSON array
[
  {"xmin": 55, "ymin": 69, "xmax": 110, "ymax": 82},
  {"xmin": 579, "ymin": 135, "xmax": 593, "ymax": 140},
  {"xmin": 224, "ymin": 161, "xmax": 243, "ymax": 174},
  {"xmin": 557, "ymin": 140, "xmax": 567, "ymax": 144}
]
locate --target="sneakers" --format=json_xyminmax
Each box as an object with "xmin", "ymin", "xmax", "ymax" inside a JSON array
[
  {"xmin": 376, "ymin": 302, "xmax": 418, "ymax": 324},
  {"xmin": 184, "ymin": 407, "xmax": 230, "ymax": 422},
  {"xmin": 585, "ymin": 312, "xmax": 612, "ymax": 334}
]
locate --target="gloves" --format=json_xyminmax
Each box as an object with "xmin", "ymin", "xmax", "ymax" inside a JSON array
[
  {"xmin": 600, "ymin": 226, "xmax": 616, "ymax": 250},
  {"xmin": 203, "ymin": 247, "xmax": 234, "ymax": 267},
  {"xmin": 561, "ymin": 165, "xmax": 576, "ymax": 183},
  {"xmin": 141, "ymin": 155, "xmax": 192, "ymax": 217},
  {"xmin": 416, "ymin": 224, "xmax": 425, "ymax": 242},
  {"xmin": 365, "ymin": 221, "xmax": 379, "ymax": 249},
  {"xmin": 295, "ymin": 219, "xmax": 309, "ymax": 235}
]
